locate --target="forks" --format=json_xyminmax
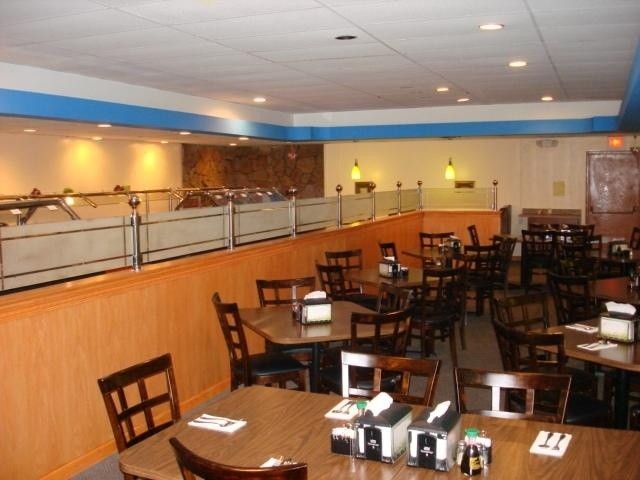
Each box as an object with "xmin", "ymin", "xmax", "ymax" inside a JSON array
[{"xmin": 193, "ymin": 420, "xmax": 229, "ymax": 427}]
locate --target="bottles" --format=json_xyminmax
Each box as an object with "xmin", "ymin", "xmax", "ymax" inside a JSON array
[
  {"xmin": 627, "ymin": 269, "xmax": 636, "ymax": 290},
  {"xmin": 456, "ymin": 428, "xmax": 492, "ymax": 477},
  {"xmin": 293, "ymin": 300, "xmax": 301, "ymax": 323},
  {"xmin": 348, "ymin": 401, "xmax": 367, "ymax": 458}
]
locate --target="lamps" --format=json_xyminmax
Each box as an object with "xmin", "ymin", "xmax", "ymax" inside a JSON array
[
  {"xmin": 352, "ymin": 159, "xmax": 360, "ymax": 180},
  {"xmin": 445, "ymin": 157, "xmax": 455, "ymax": 179}
]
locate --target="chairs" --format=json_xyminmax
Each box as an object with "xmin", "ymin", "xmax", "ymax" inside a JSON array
[
  {"xmin": 169, "ymin": 437, "xmax": 308, "ymax": 480},
  {"xmin": 98, "ymin": 352, "xmax": 182, "ymax": 480}
]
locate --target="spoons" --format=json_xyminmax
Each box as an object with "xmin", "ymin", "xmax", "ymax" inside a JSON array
[
  {"xmin": 332, "ymin": 401, "xmax": 352, "ymax": 413},
  {"xmin": 539, "ymin": 432, "xmax": 553, "ymax": 447},
  {"xmin": 552, "ymin": 435, "xmax": 565, "ymax": 450}
]
